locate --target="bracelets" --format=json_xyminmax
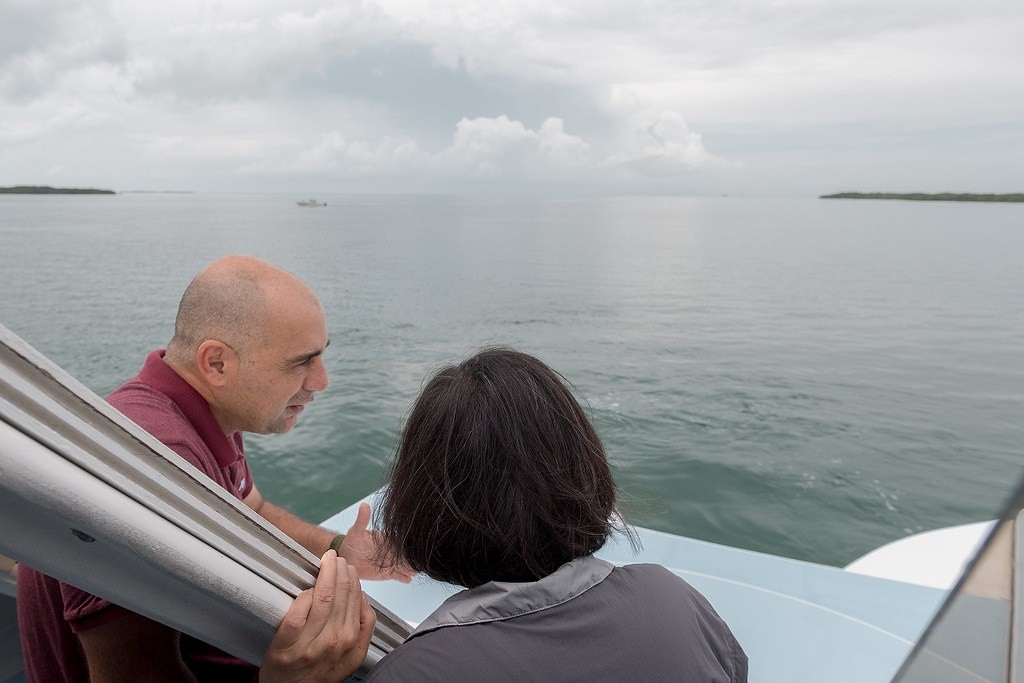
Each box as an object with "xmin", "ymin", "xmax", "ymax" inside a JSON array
[{"xmin": 327, "ymin": 534, "xmax": 346, "ymax": 561}]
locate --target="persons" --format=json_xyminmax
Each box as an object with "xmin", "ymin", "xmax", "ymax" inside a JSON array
[
  {"xmin": 15, "ymin": 255, "xmax": 421, "ymax": 683},
  {"xmin": 258, "ymin": 346, "xmax": 749, "ymax": 683}
]
